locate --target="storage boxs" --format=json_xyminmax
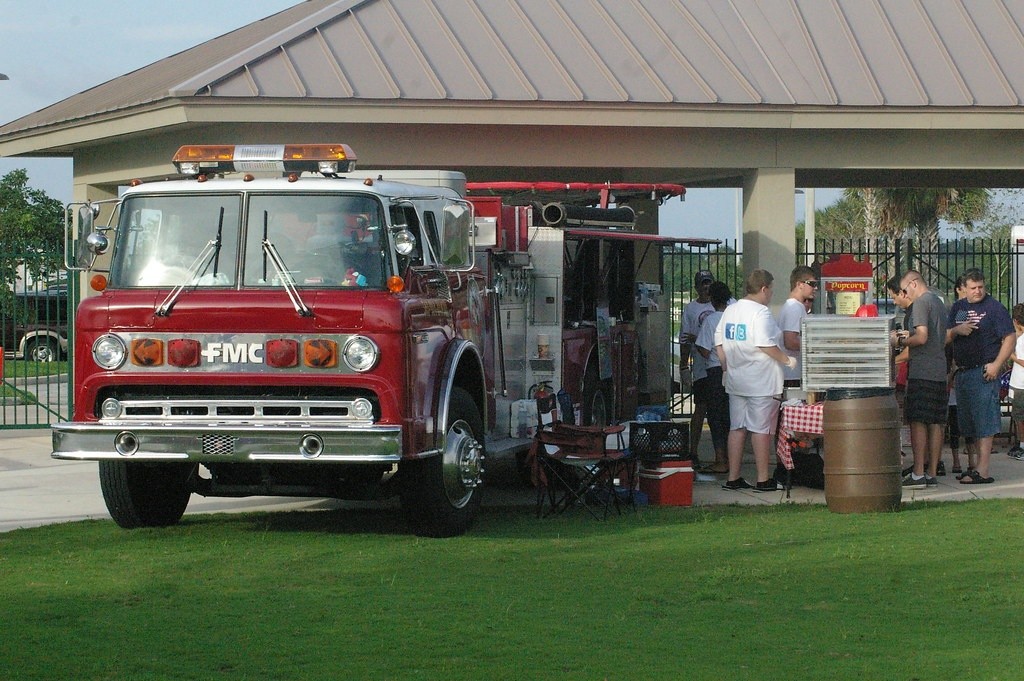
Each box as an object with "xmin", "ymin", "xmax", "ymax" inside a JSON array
[
  {"xmin": 629, "ymin": 420, "xmax": 689, "ymax": 459},
  {"xmin": 635, "ymin": 460, "xmax": 694, "ymax": 506}
]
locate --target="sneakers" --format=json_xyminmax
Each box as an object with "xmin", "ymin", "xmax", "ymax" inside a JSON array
[
  {"xmin": 926, "ymin": 477, "xmax": 938, "ymax": 487},
  {"xmin": 902, "ymin": 474, "xmax": 927, "ymax": 489}
]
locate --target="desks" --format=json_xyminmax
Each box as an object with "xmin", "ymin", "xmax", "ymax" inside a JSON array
[{"xmin": 774, "ymin": 403, "xmax": 906, "ymax": 499}]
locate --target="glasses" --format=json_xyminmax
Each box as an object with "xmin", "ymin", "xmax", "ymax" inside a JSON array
[
  {"xmin": 804, "ymin": 297, "xmax": 814, "ymax": 304},
  {"xmin": 802, "ymin": 281, "xmax": 818, "ymax": 287},
  {"xmin": 903, "ymin": 278, "xmax": 918, "ymax": 294}
]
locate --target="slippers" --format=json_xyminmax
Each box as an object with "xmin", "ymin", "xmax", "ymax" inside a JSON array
[
  {"xmin": 956, "ymin": 467, "xmax": 973, "ymax": 480},
  {"xmin": 960, "ymin": 471, "xmax": 995, "ymax": 484}
]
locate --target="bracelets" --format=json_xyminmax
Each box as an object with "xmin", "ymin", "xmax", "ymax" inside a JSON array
[
  {"xmin": 786, "ymin": 357, "xmax": 791, "ymax": 365},
  {"xmin": 898, "ymin": 337, "xmax": 904, "ymax": 346}
]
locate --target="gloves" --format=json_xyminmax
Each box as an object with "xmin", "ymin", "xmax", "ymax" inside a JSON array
[
  {"xmin": 681, "ymin": 368, "xmax": 692, "ymax": 385},
  {"xmin": 786, "ymin": 355, "xmax": 797, "ymax": 370},
  {"xmin": 722, "ymin": 370, "xmax": 726, "ymax": 387}
]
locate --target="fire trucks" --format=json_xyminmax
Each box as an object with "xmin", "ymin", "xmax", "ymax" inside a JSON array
[{"xmin": 50, "ymin": 143, "xmax": 685, "ymax": 539}]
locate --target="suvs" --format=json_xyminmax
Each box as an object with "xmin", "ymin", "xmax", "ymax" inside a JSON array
[{"xmin": 0, "ymin": 290, "xmax": 68, "ymax": 363}]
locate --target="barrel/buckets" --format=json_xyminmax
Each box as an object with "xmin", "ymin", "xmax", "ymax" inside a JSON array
[{"xmin": 823, "ymin": 386, "xmax": 902, "ymax": 511}]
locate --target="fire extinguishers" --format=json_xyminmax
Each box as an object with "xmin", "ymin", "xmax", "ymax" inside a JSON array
[{"xmin": 528, "ymin": 380, "xmax": 554, "ymax": 440}]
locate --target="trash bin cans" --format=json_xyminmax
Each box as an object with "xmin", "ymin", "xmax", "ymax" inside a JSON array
[{"xmin": 824, "ymin": 387, "xmax": 902, "ymax": 514}]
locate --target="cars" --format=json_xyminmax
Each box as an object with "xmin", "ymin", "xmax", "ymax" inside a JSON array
[
  {"xmin": 873, "ymin": 298, "xmax": 895, "ymax": 316},
  {"xmin": 44, "ymin": 267, "xmax": 67, "ymax": 289}
]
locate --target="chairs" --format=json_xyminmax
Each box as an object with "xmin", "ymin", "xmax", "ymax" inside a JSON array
[
  {"xmin": 525, "ymin": 421, "xmax": 635, "ymax": 523},
  {"xmin": 993, "ymin": 369, "xmax": 1018, "ymax": 445}
]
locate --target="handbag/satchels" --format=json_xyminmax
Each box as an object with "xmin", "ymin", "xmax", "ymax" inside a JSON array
[{"xmin": 774, "ymin": 452, "xmax": 825, "ymax": 489}]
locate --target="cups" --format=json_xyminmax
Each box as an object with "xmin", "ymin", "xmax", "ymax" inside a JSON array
[{"xmin": 537, "ymin": 334, "xmax": 550, "ymax": 359}]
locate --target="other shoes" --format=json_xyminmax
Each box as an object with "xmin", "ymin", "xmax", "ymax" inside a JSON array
[
  {"xmin": 723, "ymin": 478, "xmax": 753, "ymax": 490},
  {"xmin": 1008, "ymin": 446, "xmax": 1024, "ymax": 460},
  {"xmin": 937, "ymin": 461, "xmax": 945, "ymax": 476},
  {"xmin": 755, "ymin": 478, "xmax": 784, "ymax": 491},
  {"xmin": 697, "ymin": 460, "xmax": 727, "ymax": 473},
  {"xmin": 902, "ymin": 463, "xmax": 928, "ymax": 476},
  {"xmin": 953, "ymin": 465, "xmax": 962, "ymax": 473}
]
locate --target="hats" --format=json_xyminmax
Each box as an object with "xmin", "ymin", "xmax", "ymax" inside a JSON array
[{"xmin": 695, "ymin": 271, "xmax": 714, "ymax": 289}]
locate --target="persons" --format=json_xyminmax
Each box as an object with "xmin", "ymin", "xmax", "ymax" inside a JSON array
[
  {"xmin": 715, "ymin": 269, "xmax": 798, "ymax": 492},
  {"xmin": 679, "ymin": 270, "xmax": 737, "ymax": 465},
  {"xmin": 887, "ymin": 270, "xmax": 946, "ymax": 492},
  {"xmin": 946, "ymin": 267, "xmax": 1015, "ymax": 486},
  {"xmin": 682, "ymin": 280, "xmax": 734, "ymax": 475},
  {"xmin": 777, "ymin": 266, "xmax": 819, "ymax": 488},
  {"xmin": 1007, "ymin": 303, "xmax": 1024, "ymax": 460}
]
